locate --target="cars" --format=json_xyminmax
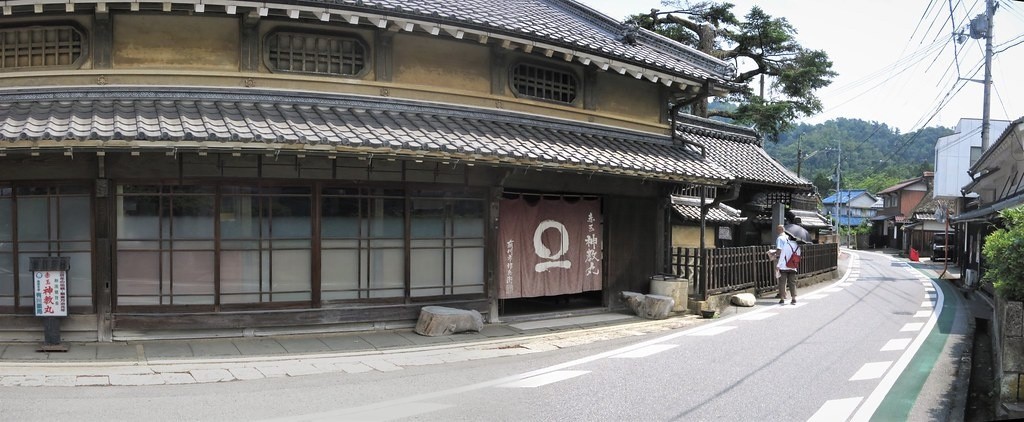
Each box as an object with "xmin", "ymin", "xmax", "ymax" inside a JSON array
[{"xmin": 930, "ymin": 233, "xmax": 956, "ymax": 262}]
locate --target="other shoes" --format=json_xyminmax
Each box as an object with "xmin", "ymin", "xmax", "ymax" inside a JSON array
[
  {"xmin": 779, "ymin": 299, "xmax": 784, "ymax": 304},
  {"xmin": 791, "ymin": 300, "xmax": 796, "ymax": 305},
  {"xmin": 775, "ymin": 293, "xmax": 787, "ymax": 299}
]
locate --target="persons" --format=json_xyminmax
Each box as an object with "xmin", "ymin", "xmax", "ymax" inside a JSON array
[
  {"xmin": 775, "ymin": 225, "xmax": 788, "ymax": 299},
  {"xmin": 776, "ymin": 235, "xmax": 801, "ymax": 305}
]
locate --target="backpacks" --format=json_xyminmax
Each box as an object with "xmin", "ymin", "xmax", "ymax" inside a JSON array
[{"xmin": 785, "ymin": 243, "xmax": 801, "ymax": 268}]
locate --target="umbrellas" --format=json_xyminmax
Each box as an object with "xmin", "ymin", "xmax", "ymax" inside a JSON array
[{"xmin": 783, "ymin": 224, "xmax": 813, "ymax": 244}]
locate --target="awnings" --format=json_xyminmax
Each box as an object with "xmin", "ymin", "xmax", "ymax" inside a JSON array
[{"xmin": 869, "ymin": 213, "xmax": 901, "ymax": 221}]
